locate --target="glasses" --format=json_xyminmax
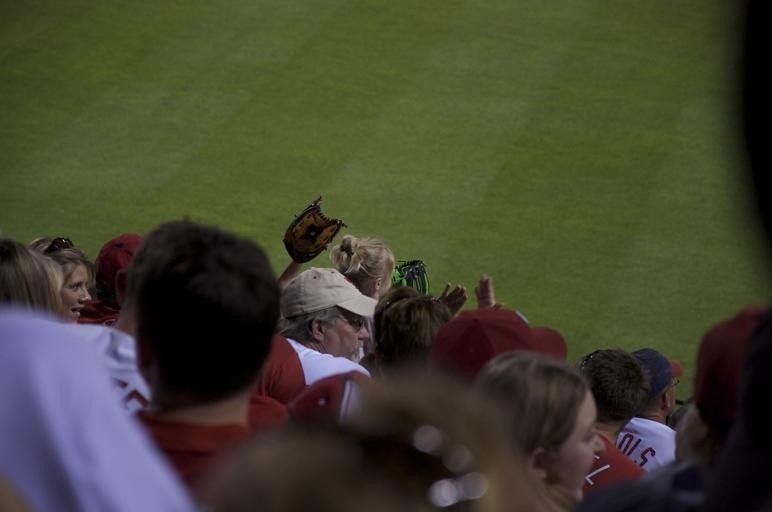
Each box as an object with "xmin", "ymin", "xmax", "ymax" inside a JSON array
[{"xmin": 41, "ymin": 237, "xmax": 73, "ymax": 257}]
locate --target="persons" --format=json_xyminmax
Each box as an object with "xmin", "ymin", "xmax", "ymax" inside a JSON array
[
  {"xmin": 260, "ymin": 199, "xmax": 567, "ymax": 403},
  {"xmin": 130, "ymin": 215, "xmax": 294, "ymax": 486},
  {"xmin": 574, "ymin": 299, "xmax": 772, "ymax": 511},
  {"xmin": 1, "ymin": 232, "xmax": 143, "ymax": 332},
  {"xmin": 211, "ymin": 383, "xmax": 561, "ymax": 512},
  {"xmin": 472, "ymin": 351, "xmax": 601, "ymax": 508},
  {"xmin": 0, "ymin": 318, "xmax": 194, "ymax": 511}
]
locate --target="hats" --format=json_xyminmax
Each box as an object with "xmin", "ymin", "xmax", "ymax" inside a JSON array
[
  {"xmin": 280, "ymin": 266, "xmax": 379, "ymax": 319},
  {"xmin": 94, "ymin": 233, "xmax": 144, "ymax": 288},
  {"xmin": 631, "ymin": 349, "xmax": 683, "ymax": 399},
  {"xmin": 426, "ymin": 308, "xmax": 567, "ymax": 390}
]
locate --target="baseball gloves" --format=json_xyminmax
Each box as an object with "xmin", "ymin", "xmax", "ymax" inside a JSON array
[
  {"xmin": 282, "ymin": 195, "xmax": 346, "ymax": 262},
  {"xmin": 391, "ymin": 259, "xmax": 429, "ymax": 295}
]
